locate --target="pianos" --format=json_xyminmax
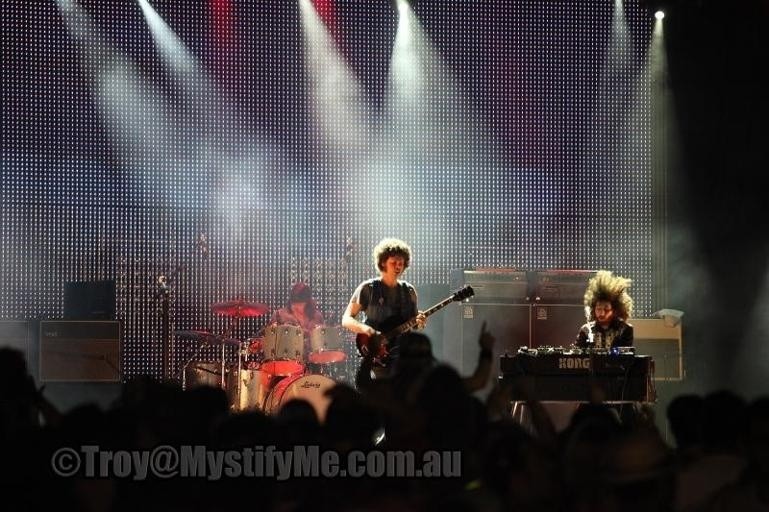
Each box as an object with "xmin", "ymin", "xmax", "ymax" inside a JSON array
[{"xmin": 497, "ymin": 345, "xmax": 658, "ymax": 405}]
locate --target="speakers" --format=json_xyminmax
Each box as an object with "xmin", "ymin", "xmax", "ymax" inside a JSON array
[
  {"xmin": 442, "ymin": 299, "xmax": 531, "ymax": 375},
  {"xmin": 62, "ymin": 279, "xmax": 116, "ymax": 318},
  {"xmin": 533, "ymin": 303, "xmax": 586, "ymax": 347},
  {"xmin": 629, "ymin": 319, "xmax": 683, "ymax": 382},
  {"xmin": 39, "ymin": 318, "xmax": 127, "ymax": 384}
]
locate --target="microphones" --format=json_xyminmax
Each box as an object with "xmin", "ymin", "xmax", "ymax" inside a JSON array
[{"xmin": 198, "ymin": 229, "xmax": 213, "ymax": 261}]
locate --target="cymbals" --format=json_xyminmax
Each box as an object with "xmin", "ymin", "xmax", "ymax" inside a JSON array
[
  {"xmin": 211, "ymin": 301, "xmax": 267, "ymax": 316},
  {"xmin": 174, "ymin": 330, "xmax": 239, "ymax": 346}
]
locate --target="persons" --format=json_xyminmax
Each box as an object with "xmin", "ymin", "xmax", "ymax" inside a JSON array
[
  {"xmin": 246, "ymin": 282, "xmax": 327, "ymax": 375},
  {"xmin": 339, "ymin": 237, "xmax": 427, "ymax": 393},
  {"xmin": 570, "ymin": 269, "xmax": 634, "ymax": 353}
]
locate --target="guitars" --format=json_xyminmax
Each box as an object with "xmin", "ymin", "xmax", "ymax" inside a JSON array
[{"xmin": 356, "ymin": 284, "xmax": 474, "ymax": 366}]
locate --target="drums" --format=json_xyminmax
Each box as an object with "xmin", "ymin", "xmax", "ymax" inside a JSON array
[
  {"xmin": 265, "ymin": 373, "xmax": 337, "ymax": 423},
  {"xmin": 308, "ymin": 324, "xmax": 346, "ymax": 364},
  {"xmin": 261, "ymin": 321, "xmax": 305, "ymax": 377},
  {"xmin": 227, "ymin": 368, "xmax": 272, "ymax": 413},
  {"xmin": 179, "ymin": 360, "xmax": 227, "ymax": 388}
]
